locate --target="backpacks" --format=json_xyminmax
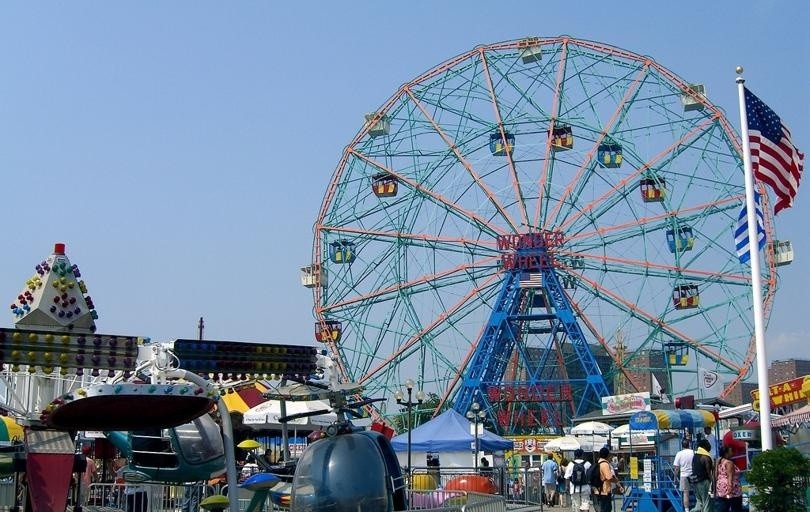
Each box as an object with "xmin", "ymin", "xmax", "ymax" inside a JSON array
[
  {"xmin": 571, "ymin": 460, "xmax": 586, "ymax": 485},
  {"xmin": 586, "ymin": 461, "xmax": 609, "ymax": 487}
]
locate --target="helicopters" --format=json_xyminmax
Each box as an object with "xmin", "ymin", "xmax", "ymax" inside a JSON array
[
  {"xmin": 101, "ymin": 414, "xmax": 226, "ymax": 483},
  {"xmin": 277, "ymin": 397, "xmax": 409, "ymax": 512}
]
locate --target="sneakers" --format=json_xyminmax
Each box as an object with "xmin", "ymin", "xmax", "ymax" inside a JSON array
[{"xmin": 548, "ymin": 500, "xmax": 553, "ymax": 507}]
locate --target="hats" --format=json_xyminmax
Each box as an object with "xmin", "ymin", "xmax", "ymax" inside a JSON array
[{"xmin": 82, "ymin": 445, "xmax": 92, "ymax": 456}]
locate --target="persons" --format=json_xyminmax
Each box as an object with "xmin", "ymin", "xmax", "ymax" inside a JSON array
[
  {"xmin": 236, "ymin": 449, "xmax": 291, "ymax": 484},
  {"xmin": 72, "ymin": 444, "xmax": 99, "ymax": 505},
  {"xmin": 182, "ymin": 480, "xmax": 203, "ymax": 512},
  {"xmin": 681, "ymin": 292, "xmax": 686, "ymax": 297},
  {"xmin": 478, "ymin": 425, "xmax": 749, "ymax": 512}
]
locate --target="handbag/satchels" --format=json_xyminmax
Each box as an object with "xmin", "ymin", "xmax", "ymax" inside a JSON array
[
  {"xmin": 557, "ymin": 472, "xmax": 565, "ymax": 483},
  {"xmin": 689, "ymin": 475, "xmax": 697, "ymax": 484}
]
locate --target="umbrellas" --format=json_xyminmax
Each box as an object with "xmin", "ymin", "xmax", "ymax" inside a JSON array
[
  {"xmin": 236, "ymin": 399, "xmax": 337, "ymax": 465},
  {"xmin": 570, "ymin": 421, "xmax": 656, "ymax": 464}
]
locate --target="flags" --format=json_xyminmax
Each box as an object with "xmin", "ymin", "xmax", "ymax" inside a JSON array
[{"xmin": 734, "ymin": 85, "xmax": 808, "ymax": 265}]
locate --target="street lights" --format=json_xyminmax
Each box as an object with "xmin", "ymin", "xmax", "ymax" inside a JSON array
[
  {"xmin": 395, "ymin": 379, "xmax": 424, "ymax": 492},
  {"xmin": 468, "ymin": 402, "xmax": 485, "ymax": 470}
]
